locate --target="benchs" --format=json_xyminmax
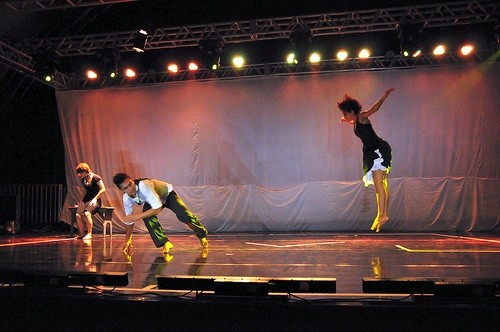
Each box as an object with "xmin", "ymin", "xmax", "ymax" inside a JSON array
[{"xmin": 68, "ymin": 205, "xmax": 115, "ymax": 239}]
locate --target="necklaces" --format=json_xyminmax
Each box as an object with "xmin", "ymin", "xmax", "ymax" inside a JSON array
[
  {"xmin": 112, "ymin": 172, "xmax": 208, "ymax": 254},
  {"xmin": 84, "ymin": 174, "xmax": 92, "ymax": 178},
  {"xmin": 74, "ymin": 163, "xmax": 106, "ymax": 240}
]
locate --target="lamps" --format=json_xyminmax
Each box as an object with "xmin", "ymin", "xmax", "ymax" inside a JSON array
[{"xmin": 132, "ymin": 29, "xmax": 149, "ymax": 54}]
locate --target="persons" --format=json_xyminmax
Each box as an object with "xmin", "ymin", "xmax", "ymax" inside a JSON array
[{"xmin": 336, "ymin": 88, "xmax": 396, "ymax": 232}]
